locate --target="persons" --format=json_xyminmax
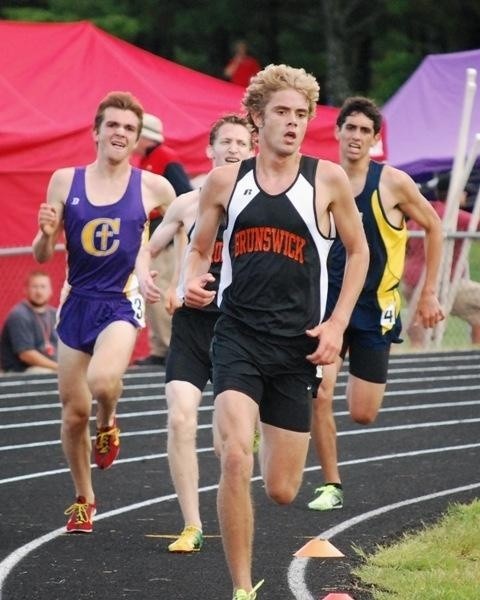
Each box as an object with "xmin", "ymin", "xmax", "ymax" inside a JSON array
[
  {"xmin": 137, "ymin": 110, "xmax": 263, "ymax": 554},
  {"xmin": 401, "ymin": 178, "xmax": 480, "ymax": 351},
  {"xmin": 180, "ymin": 60, "xmax": 372, "ymax": 600},
  {"xmin": 127, "ymin": 113, "xmax": 195, "ymax": 369},
  {"xmin": 305, "ymin": 93, "xmax": 446, "ymax": 511},
  {"xmin": 1, "ymin": 270, "xmax": 60, "ymax": 375},
  {"xmin": 32, "ymin": 91, "xmax": 190, "ymax": 535}
]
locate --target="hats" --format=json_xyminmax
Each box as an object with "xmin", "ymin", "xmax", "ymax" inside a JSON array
[{"xmin": 141, "ymin": 113, "xmax": 164, "ymax": 144}]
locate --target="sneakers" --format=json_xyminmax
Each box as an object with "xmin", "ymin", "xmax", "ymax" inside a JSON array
[
  {"xmin": 67, "ymin": 498, "xmax": 98, "ymax": 534},
  {"xmin": 95, "ymin": 414, "xmax": 119, "ymax": 471},
  {"xmin": 308, "ymin": 486, "xmax": 344, "ymax": 511},
  {"xmin": 167, "ymin": 529, "xmax": 204, "ymax": 553},
  {"xmin": 134, "ymin": 356, "xmax": 164, "ymax": 365}
]
